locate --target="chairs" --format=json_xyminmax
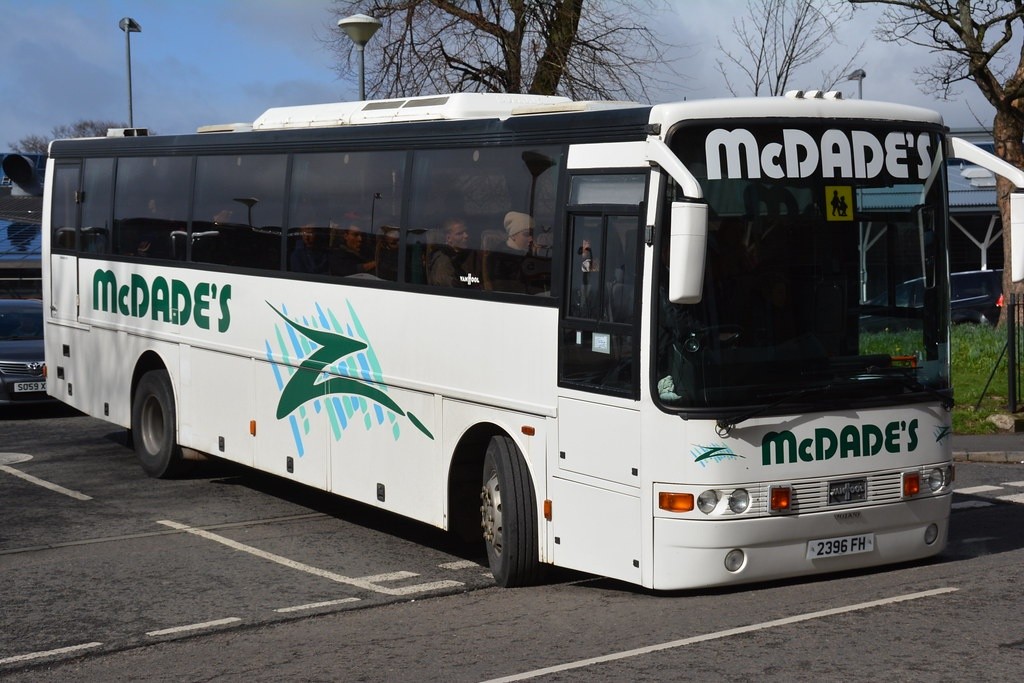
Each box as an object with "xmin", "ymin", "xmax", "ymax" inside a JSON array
[{"xmin": 325, "ymin": 219, "xmax": 506, "ymax": 294}]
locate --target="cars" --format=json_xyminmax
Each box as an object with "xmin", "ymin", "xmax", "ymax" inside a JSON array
[{"xmin": 0, "ymin": 299, "xmax": 61, "ymax": 419}]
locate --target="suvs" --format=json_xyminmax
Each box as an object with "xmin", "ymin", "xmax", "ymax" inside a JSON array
[{"xmin": 855, "ymin": 269, "xmax": 1003, "ymax": 335}]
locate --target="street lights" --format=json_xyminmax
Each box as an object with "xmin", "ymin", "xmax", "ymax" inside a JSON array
[
  {"xmin": 520, "ymin": 150, "xmax": 557, "ymax": 256},
  {"xmin": 370, "ymin": 192, "xmax": 383, "ymax": 233},
  {"xmin": 847, "ymin": 68, "xmax": 866, "ymax": 100},
  {"xmin": 119, "ymin": 16, "xmax": 141, "ymax": 128},
  {"xmin": 337, "ymin": 12, "xmax": 383, "ymax": 101},
  {"xmin": 232, "ymin": 197, "xmax": 260, "ymax": 227}
]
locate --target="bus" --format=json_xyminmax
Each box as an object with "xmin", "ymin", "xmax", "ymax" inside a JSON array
[{"xmin": 40, "ymin": 91, "xmax": 1024, "ymax": 591}]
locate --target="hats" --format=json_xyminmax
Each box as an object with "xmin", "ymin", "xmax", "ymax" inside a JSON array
[{"xmin": 503, "ymin": 212, "xmax": 536, "ymax": 237}]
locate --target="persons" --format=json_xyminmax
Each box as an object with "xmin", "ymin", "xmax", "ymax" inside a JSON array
[
  {"xmin": 488, "ymin": 211, "xmax": 551, "ymax": 294},
  {"xmin": 112, "ymin": 199, "xmax": 400, "ymax": 282},
  {"xmin": 714, "ymin": 216, "xmax": 751, "ymax": 321},
  {"xmin": 429, "ymin": 217, "xmax": 473, "ymax": 288},
  {"xmin": 752, "ymin": 275, "xmax": 799, "ymax": 346}
]
